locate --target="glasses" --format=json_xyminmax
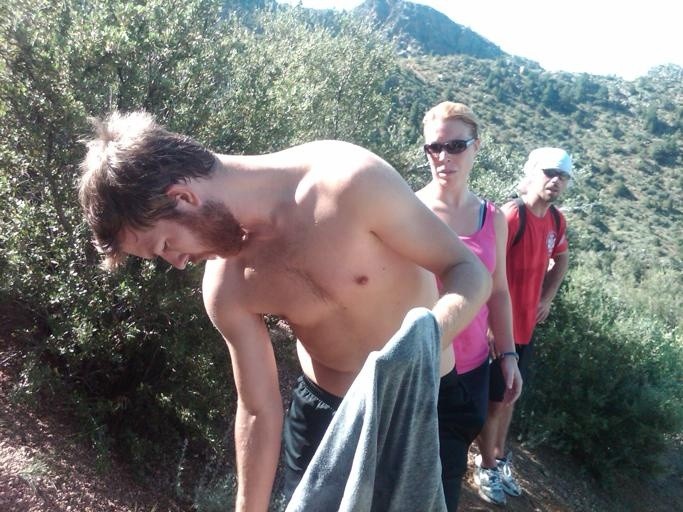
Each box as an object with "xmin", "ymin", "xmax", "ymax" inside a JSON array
[
  {"xmin": 422, "ymin": 137, "xmax": 474, "ymax": 155},
  {"xmin": 541, "ymin": 166, "xmax": 571, "ymax": 180}
]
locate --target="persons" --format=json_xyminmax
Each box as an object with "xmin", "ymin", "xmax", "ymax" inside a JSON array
[
  {"xmin": 78, "ymin": 109, "xmax": 494, "ymax": 512},
  {"xmin": 462, "ymin": 148, "xmax": 573, "ymax": 503},
  {"xmin": 411, "ymin": 100, "xmax": 525, "ymax": 510}
]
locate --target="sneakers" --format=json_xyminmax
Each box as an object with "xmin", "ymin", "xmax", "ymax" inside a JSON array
[
  {"xmin": 474, "ymin": 464, "xmax": 509, "ymax": 507},
  {"xmin": 493, "ymin": 454, "xmax": 522, "ymax": 498}
]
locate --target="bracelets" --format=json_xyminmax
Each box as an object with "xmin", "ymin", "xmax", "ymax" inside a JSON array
[{"xmin": 497, "ymin": 352, "xmax": 519, "ymax": 360}]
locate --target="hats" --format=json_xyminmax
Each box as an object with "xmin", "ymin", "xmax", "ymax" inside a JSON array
[{"xmin": 525, "ymin": 148, "xmax": 577, "ymax": 181}]
